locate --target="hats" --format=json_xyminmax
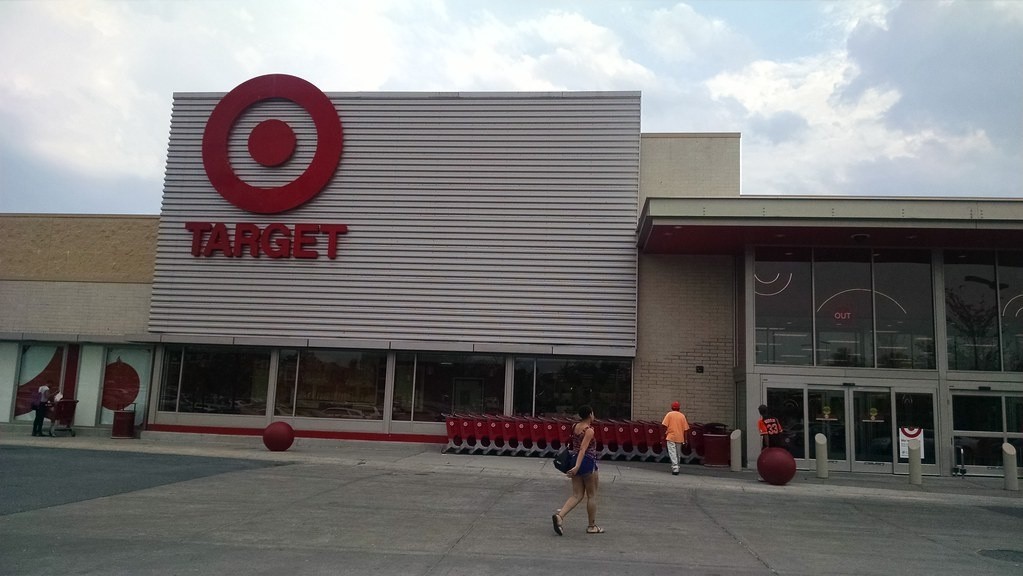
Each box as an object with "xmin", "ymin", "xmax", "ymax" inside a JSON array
[{"xmin": 672, "ymin": 401, "xmax": 680, "ymax": 408}]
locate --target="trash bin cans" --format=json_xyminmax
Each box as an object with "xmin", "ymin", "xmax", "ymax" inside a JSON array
[
  {"xmin": 111, "ymin": 402, "xmax": 136, "ymax": 439},
  {"xmin": 703, "ymin": 421, "xmax": 730, "ymax": 467}
]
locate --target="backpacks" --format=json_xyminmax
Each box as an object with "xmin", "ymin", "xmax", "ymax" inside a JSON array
[{"xmin": 31, "ymin": 392, "xmax": 42, "ymax": 408}]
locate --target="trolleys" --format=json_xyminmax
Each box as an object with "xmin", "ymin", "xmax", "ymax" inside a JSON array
[
  {"xmin": 441, "ymin": 411, "xmax": 731, "ymax": 466},
  {"xmin": 43, "ymin": 396, "xmax": 79, "ymax": 438}
]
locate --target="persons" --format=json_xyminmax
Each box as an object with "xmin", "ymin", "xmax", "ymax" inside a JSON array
[
  {"xmin": 31, "ymin": 380, "xmax": 56, "ymax": 437},
  {"xmin": 757, "ymin": 404, "xmax": 783, "ymax": 482},
  {"xmin": 662, "ymin": 401, "xmax": 690, "ymax": 475},
  {"xmin": 551, "ymin": 405, "xmax": 606, "ymax": 536}
]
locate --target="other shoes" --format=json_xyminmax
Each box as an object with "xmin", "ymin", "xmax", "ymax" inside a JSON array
[
  {"xmin": 758, "ymin": 477, "xmax": 765, "ymax": 482},
  {"xmin": 38, "ymin": 433, "xmax": 45, "ymax": 436},
  {"xmin": 32, "ymin": 432, "xmax": 37, "ymax": 435}
]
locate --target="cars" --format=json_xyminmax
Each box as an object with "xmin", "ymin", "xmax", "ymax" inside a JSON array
[
  {"xmin": 784, "ymin": 418, "xmax": 1023, "ymax": 462},
  {"xmin": 160, "ymin": 384, "xmax": 401, "ymax": 420}
]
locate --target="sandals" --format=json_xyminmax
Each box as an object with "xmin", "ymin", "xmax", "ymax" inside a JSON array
[
  {"xmin": 586, "ymin": 525, "xmax": 605, "ymax": 533},
  {"xmin": 552, "ymin": 513, "xmax": 564, "ymax": 536}
]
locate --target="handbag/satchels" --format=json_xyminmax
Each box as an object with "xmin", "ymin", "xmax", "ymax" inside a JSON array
[
  {"xmin": 567, "ymin": 450, "xmax": 598, "ymax": 474},
  {"xmin": 553, "ymin": 422, "xmax": 581, "ymax": 474},
  {"xmin": 54, "ymin": 392, "xmax": 63, "ymax": 402}
]
